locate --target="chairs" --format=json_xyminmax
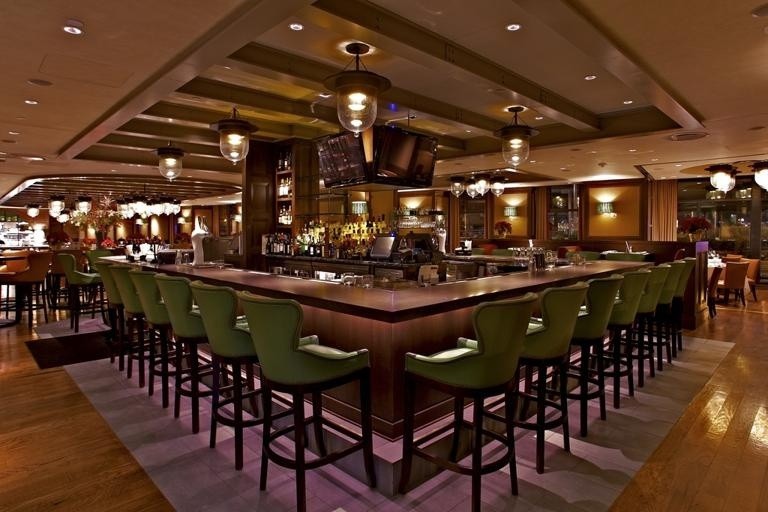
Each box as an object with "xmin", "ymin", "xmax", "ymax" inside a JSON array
[
  {"xmin": 473, "ymin": 281, "xmax": 590, "ymax": 473},
  {"xmin": 455, "ymin": 246, "xmax": 650, "ymax": 260},
  {"xmin": 735, "ymin": 258, "xmax": 761, "ymax": 302},
  {"xmin": 85, "ymin": 249, "xmax": 113, "ymax": 272},
  {"xmin": 188, "ymin": 279, "xmax": 311, "ymax": 471},
  {"xmin": 94, "ymin": 262, "xmax": 174, "ymax": 373},
  {"xmin": 238, "ymin": 291, "xmax": 376, "ymax": 511},
  {"xmin": 58, "ymin": 252, "xmax": 105, "ymax": 334},
  {"xmin": 707, "ymin": 267, "xmax": 722, "ymax": 319},
  {"xmin": 722, "ymin": 253, "xmax": 743, "ymax": 263},
  {"xmin": 153, "ymin": 271, "xmax": 259, "ymax": 433},
  {"xmin": 399, "ymin": 291, "xmax": 537, "ymax": 511},
  {"xmin": 636, "ymin": 255, "xmax": 704, "ymax": 360},
  {"xmin": 129, "ymin": 270, "xmax": 231, "ymax": 409},
  {"xmin": 719, "ymin": 261, "xmax": 750, "ymax": 307},
  {"xmin": 638, "ymin": 260, "xmax": 689, "ymax": 372},
  {"xmin": 111, "ymin": 265, "xmax": 194, "ymax": 387},
  {"xmin": 522, "ymin": 273, "xmax": 626, "ymax": 438},
  {"xmin": 0, "ymin": 250, "xmax": 52, "ymax": 327},
  {"xmin": 621, "ymin": 264, "xmax": 672, "ymax": 386},
  {"xmin": 588, "ymin": 267, "xmax": 654, "ymax": 407}
]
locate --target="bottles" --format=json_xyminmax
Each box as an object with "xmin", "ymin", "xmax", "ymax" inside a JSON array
[
  {"xmin": 276, "ymin": 204, "xmax": 292, "ymax": 226},
  {"xmin": 274, "ymin": 148, "xmax": 291, "ymax": 171},
  {"xmin": 276, "ymin": 176, "xmax": 293, "ymax": 199},
  {"xmin": 264, "ymin": 213, "xmax": 387, "ymax": 264},
  {"xmin": 385, "ymin": 207, "xmax": 445, "ymax": 233}
]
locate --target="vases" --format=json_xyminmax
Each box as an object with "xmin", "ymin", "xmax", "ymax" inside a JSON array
[
  {"xmin": 688, "ymin": 233, "xmax": 702, "ymax": 242},
  {"xmin": 95, "ymin": 231, "xmax": 105, "ymax": 248},
  {"xmin": 498, "ymin": 231, "xmax": 507, "ymax": 240}
]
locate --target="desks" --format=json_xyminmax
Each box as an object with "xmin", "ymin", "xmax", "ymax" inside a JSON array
[{"xmin": 707, "ymin": 261, "xmax": 726, "ymax": 287}]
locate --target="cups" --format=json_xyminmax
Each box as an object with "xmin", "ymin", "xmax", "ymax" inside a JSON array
[{"xmin": 508, "ymin": 246, "xmax": 589, "ymax": 273}]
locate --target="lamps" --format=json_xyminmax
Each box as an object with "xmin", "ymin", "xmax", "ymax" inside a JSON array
[
  {"xmin": 475, "ymin": 175, "xmax": 491, "ymax": 197},
  {"xmin": 210, "ymin": 108, "xmax": 260, "ymax": 166},
  {"xmin": 502, "ymin": 204, "xmax": 520, "ymax": 219},
  {"xmin": 493, "ymin": 105, "xmax": 541, "ymax": 170},
  {"xmin": 318, "ymin": 41, "xmax": 394, "ymax": 138},
  {"xmin": 752, "ymin": 162, "xmax": 768, "ymax": 193},
  {"xmin": 119, "ymin": 193, "xmax": 181, "ymax": 227},
  {"xmin": 596, "ymin": 201, "xmax": 616, "ymax": 216},
  {"xmin": 154, "ymin": 140, "xmax": 185, "ymax": 184},
  {"xmin": 487, "ymin": 176, "xmax": 505, "ymax": 198},
  {"xmin": 708, "ymin": 167, "xmax": 732, "ymax": 192},
  {"xmin": 350, "ymin": 200, "xmax": 367, "ymax": 217},
  {"xmin": 448, "ymin": 177, "xmax": 465, "ymax": 199},
  {"xmin": 24, "ymin": 196, "xmax": 89, "ymax": 223},
  {"xmin": 722, "ymin": 172, "xmax": 736, "ymax": 194},
  {"xmin": 465, "ymin": 178, "xmax": 479, "ymax": 199}
]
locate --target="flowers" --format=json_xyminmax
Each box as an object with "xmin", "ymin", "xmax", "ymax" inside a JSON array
[
  {"xmin": 494, "ymin": 221, "xmax": 513, "ymax": 234},
  {"xmin": 674, "ymin": 216, "xmax": 713, "ymax": 233},
  {"xmin": 80, "ymin": 237, "xmax": 97, "ymax": 245},
  {"xmin": 79, "ymin": 196, "xmax": 117, "ymax": 230},
  {"xmin": 99, "ymin": 238, "xmax": 116, "ymax": 250}
]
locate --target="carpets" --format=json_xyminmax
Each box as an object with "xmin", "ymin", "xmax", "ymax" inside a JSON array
[
  {"xmin": 60, "ymin": 349, "xmax": 400, "ymax": 508},
  {"xmin": 24, "ymin": 327, "xmax": 138, "ymax": 371},
  {"xmin": 30, "ymin": 315, "xmax": 110, "ymax": 339},
  {"xmin": 400, "ymin": 328, "xmax": 735, "ymax": 508}
]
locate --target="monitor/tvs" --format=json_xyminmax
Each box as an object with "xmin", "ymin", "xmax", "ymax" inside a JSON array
[
  {"xmin": 313, "ymin": 132, "xmax": 370, "ymax": 188},
  {"xmin": 370, "ymin": 236, "xmax": 396, "ymax": 262},
  {"xmin": 371, "ymin": 125, "xmax": 439, "ymax": 188}
]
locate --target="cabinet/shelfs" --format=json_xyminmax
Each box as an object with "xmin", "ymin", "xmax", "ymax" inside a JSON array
[{"xmin": 274, "ymin": 138, "xmax": 319, "ymax": 254}]
